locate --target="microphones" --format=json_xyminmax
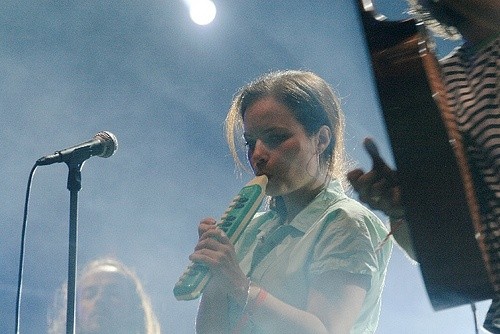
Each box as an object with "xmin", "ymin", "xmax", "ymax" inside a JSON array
[{"xmin": 36, "ymin": 130, "xmax": 118, "ymax": 166}]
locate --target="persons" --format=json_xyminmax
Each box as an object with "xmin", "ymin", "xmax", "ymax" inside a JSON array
[
  {"xmin": 196, "ymin": 69, "xmax": 394, "ymax": 334},
  {"xmin": 346, "ymin": 0, "xmax": 500, "ymax": 333},
  {"xmin": 47, "ymin": 255, "xmax": 161, "ymax": 334}
]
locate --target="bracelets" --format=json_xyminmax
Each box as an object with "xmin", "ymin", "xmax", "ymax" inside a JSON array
[
  {"xmin": 234, "ymin": 288, "xmax": 268, "ymax": 333},
  {"xmin": 240, "ymin": 277, "xmax": 253, "ymax": 311}
]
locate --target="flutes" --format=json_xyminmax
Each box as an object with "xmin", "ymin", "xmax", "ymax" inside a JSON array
[{"xmin": 173, "ymin": 175, "xmax": 269, "ymax": 300}]
